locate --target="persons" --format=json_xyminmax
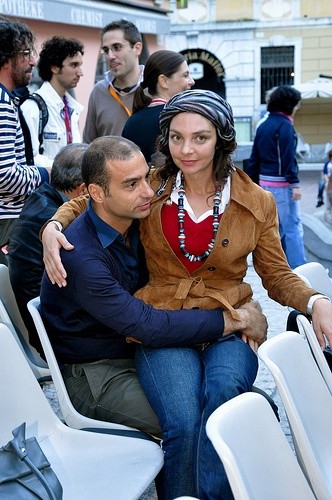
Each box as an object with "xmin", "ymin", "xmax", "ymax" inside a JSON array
[
  {"xmin": 38, "ymin": 89, "xmax": 332, "ymax": 500},
  {"xmin": 0, "ymin": 16, "xmax": 332, "ymax": 500}
]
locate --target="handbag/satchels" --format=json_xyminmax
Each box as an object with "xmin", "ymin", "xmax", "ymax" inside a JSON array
[{"xmin": 0, "ymin": 421, "xmax": 63, "ymax": 499}]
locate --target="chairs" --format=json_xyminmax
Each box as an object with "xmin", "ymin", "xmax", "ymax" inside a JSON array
[{"xmin": 0, "ymin": 262, "xmax": 332, "ymax": 500}]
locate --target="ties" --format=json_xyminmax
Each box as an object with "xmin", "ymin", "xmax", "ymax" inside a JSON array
[
  {"xmin": 13, "ymin": 96, "xmax": 33, "ymax": 166},
  {"xmin": 61, "ymin": 98, "xmax": 72, "ymax": 144}
]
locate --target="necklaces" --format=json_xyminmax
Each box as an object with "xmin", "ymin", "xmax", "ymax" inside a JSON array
[{"xmin": 178, "ymin": 174, "xmax": 221, "ymax": 262}]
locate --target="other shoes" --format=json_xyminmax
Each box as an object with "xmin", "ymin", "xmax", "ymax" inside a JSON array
[{"xmin": 316, "ymin": 200, "xmax": 323, "ymax": 208}]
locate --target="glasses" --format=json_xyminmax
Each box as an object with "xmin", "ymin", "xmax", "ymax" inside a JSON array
[
  {"xmin": 18, "ymin": 46, "xmax": 35, "ymax": 59},
  {"xmin": 98, "ymin": 42, "xmax": 135, "ymax": 55}
]
